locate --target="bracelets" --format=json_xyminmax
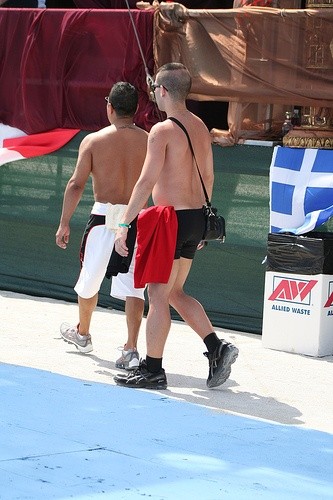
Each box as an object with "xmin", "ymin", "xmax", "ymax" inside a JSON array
[{"xmin": 118, "ymin": 224, "xmax": 131, "ymax": 227}]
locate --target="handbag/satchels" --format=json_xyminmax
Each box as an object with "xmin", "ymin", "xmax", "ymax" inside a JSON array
[{"xmin": 202, "ymin": 211, "xmax": 226, "ymax": 244}]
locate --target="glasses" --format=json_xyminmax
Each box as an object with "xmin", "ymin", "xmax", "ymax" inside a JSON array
[
  {"xmin": 104, "ymin": 96, "xmax": 114, "ymax": 109},
  {"xmin": 151, "ymin": 85, "xmax": 169, "ymax": 93}
]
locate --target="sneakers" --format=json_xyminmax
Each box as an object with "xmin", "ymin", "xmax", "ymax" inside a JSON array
[
  {"xmin": 203, "ymin": 339, "xmax": 239, "ymax": 388},
  {"xmin": 60, "ymin": 322, "xmax": 93, "ymax": 353},
  {"xmin": 113, "ymin": 358, "xmax": 167, "ymax": 390},
  {"xmin": 115, "ymin": 344, "xmax": 139, "ymax": 369}
]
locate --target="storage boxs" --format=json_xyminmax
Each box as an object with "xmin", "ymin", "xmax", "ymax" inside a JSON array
[{"xmin": 261, "ymin": 231, "xmax": 333, "ymax": 357}]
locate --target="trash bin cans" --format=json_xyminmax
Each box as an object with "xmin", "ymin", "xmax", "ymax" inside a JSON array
[{"xmin": 259, "ymin": 231, "xmax": 333, "ymax": 358}]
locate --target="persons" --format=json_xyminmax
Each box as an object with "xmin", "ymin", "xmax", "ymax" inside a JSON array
[
  {"xmin": 56, "ymin": 82, "xmax": 150, "ymax": 370},
  {"xmin": 113, "ymin": 63, "xmax": 239, "ymax": 390}
]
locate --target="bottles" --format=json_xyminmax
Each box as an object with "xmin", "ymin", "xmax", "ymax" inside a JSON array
[{"xmin": 282, "ymin": 109, "xmax": 300, "ymax": 138}]
